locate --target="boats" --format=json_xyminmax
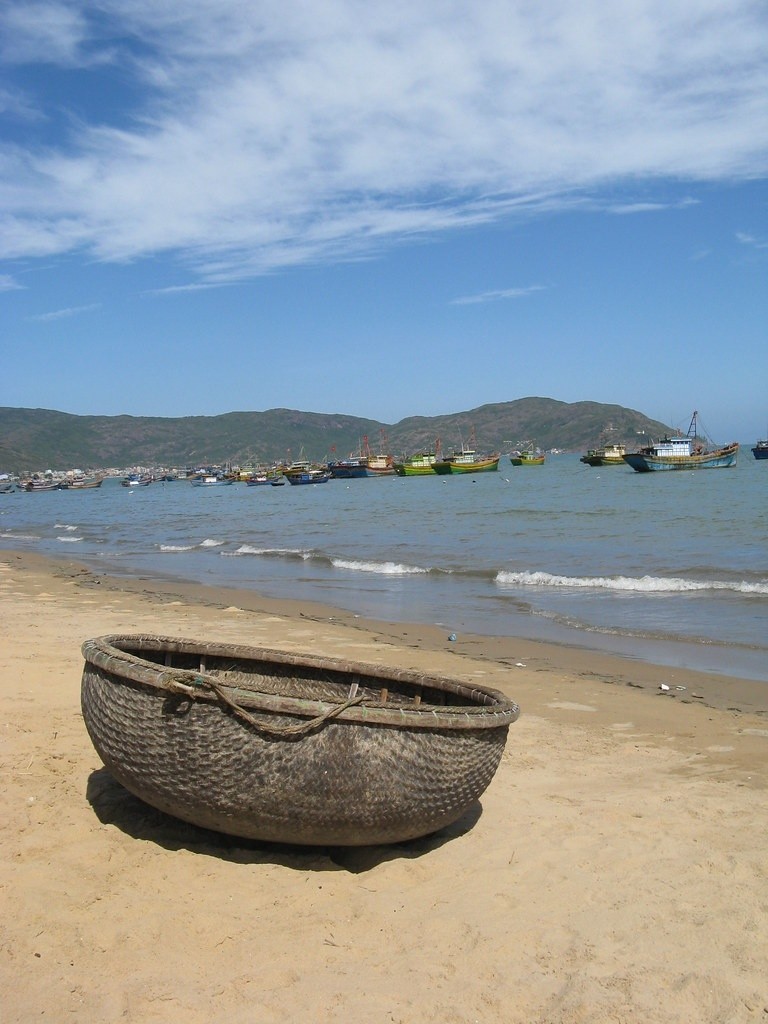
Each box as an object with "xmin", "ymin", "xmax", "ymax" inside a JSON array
[
  {"xmin": 579, "ymin": 444, "xmax": 627, "ymax": 466},
  {"xmin": 510, "ymin": 449, "xmax": 545, "ymax": 466},
  {"xmin": 0, "ymin": 449, "xmax": 501, "ymax": 494},
  {"xmin": 749, "ymin": 440, "xmax": 768, "ymax": 460},
  {"xmin": 623, "ymin": 432, "xmax": 739, "ymax": 472}
]
